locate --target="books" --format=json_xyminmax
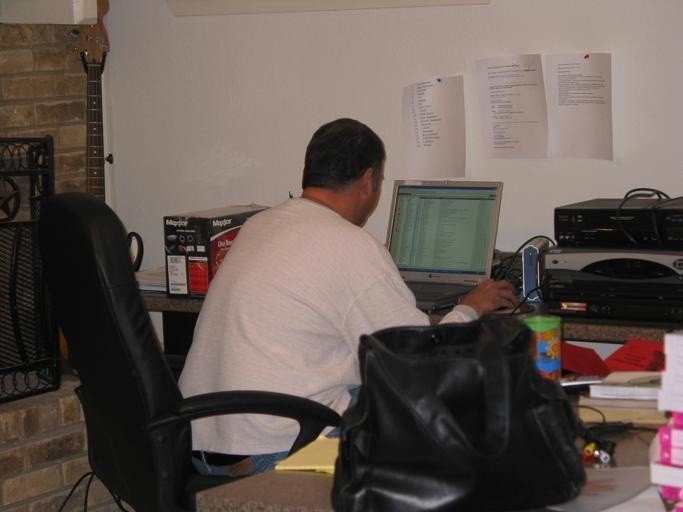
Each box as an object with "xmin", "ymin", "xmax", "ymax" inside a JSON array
[
  {"xmin": 557, "ymin": 329, "xmax": 683, "ymax": 512},
  {"xmin": 273, "ymin": 424, "xmax": 339, "ymax": 474}
]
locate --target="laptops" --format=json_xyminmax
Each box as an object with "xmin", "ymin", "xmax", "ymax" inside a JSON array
[{"xmin": 385, "ymin": 178, "xmax": 504, "ymax": 308}]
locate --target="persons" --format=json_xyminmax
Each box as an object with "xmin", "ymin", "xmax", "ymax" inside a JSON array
[{"xmin": 171, "ymin": 117, "xmax": 520, "ymax": 474}]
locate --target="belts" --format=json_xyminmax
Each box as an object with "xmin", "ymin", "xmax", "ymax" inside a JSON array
[{"xmin": 194, "ymin": 452, "xmax": 246, "ymax": 466}]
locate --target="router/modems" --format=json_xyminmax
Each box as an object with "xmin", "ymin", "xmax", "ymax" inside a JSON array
[{"xmin": 521, "ymin": 235, "xmax": 550, "ymax": 303}]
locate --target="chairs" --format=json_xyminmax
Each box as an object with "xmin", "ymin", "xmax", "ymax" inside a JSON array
[{"xmin": 36, "ymin": 194, "xmax": 343, "ymax": 511}]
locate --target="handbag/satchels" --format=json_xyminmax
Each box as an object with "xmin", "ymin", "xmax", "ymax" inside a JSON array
[{"xmin": 331, "ymin": 314, "xmax": 587, "ymax": 512}]
